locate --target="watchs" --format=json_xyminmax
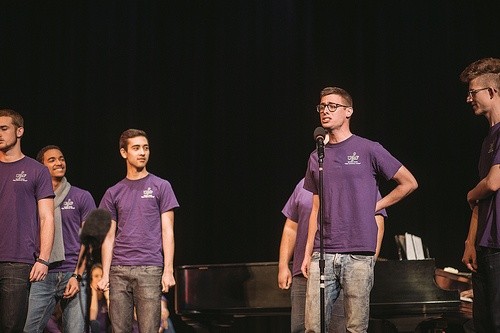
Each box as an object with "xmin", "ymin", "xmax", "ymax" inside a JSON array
[{"xmin": 71, "ymin": 273, "xmax": 82, "ymax": 282}]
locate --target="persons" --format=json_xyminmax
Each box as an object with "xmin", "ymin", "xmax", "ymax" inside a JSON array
[
  {"xmin": 277, "ymin": 174, "xmax": 387, "ymax": 333},
  {"xmin": 85, "ymin": 263, "xmax": 180, "ymax": 333},
  {"xmin": 301, "ymin": 87, "xmax": 418, "ymax": 333},
  {"xmin": 461, "ymin": 57, "xmax": 500, "ymax": 333},
  {"xmin": 95, "ymin": 129, "xmax": 181, "ymax": 332},
  {"xmin": 0, "ymin": 108, "xmax": 56, "ymax": 332},
  {"xmin": 24, "ymin": 145, "xmax": 98, "ymax": 333}
]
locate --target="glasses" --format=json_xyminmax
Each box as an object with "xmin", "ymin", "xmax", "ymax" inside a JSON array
[
  {"xmin": 318, "ymin": 103, "xmax": 348, "ymax": 112},
  {"xmin": 468, "ymin": 87, "xmax": 499, "ymax": 96}
]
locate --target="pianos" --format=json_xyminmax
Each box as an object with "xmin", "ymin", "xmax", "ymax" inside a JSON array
[{"xmin": 174, "ymin": 234, "xmax": 474, "ymax": 333}]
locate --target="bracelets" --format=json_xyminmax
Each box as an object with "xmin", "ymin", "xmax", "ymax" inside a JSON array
[{"xmin": 36, "ymin": 258, "xmax": 50, "ymax": 267}]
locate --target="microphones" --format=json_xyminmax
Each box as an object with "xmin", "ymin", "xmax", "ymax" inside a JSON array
[
  {"xmin": 79, "ymin": 209, "xmax": 112, "ymax": 248},
  {"xmin": 314, "ymin": 127, "xmax": 325, "ymax": 159}
]
